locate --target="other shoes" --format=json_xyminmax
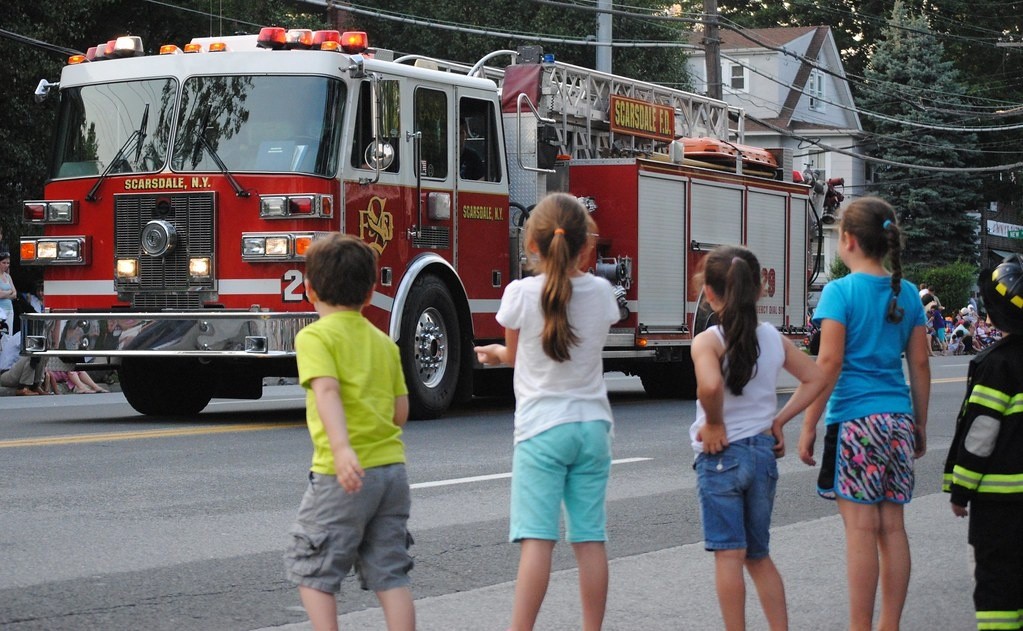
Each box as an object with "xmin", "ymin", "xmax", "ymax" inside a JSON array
[
  {"xmin": 54, "ymin": 389, "xmax": 61, "ymax": 395},
  {"xmin": 815, "ymin": 483, "xmax": 836, "ymax": 499},
  {"xmin": 91, "ymin": 386, "xmax": 110, "ymax": 393},
  {"xmin": 75, "ymin": 388, "xmax": 96, "ymax": 393}
]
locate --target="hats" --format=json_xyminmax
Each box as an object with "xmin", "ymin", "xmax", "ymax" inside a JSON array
[
  {"xmin": 967, "ymin": 304, "xmax": 974, "ymax": 309},
  {"xmin": 960, "ymin": 307, "xmax": 969, "ymax": 315}
]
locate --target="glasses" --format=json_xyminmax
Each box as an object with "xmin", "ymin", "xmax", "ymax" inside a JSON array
[{"xmin": 0, "ymin": 251, "xmax": 10, "ymax": 257}]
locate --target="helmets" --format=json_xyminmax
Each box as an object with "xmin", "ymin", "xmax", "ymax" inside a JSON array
[{"xmin": 991, "ymin": 252, "xmax": 1023, "ymax": 308}]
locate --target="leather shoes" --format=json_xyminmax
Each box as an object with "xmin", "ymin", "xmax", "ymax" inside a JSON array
[
  {"xmin": 15, "ymin": 388, "xmax": 39, "ymax": 395},
  {"xmin": 36, "ymin": 388, "xmax": 50, "ymax": 395}
]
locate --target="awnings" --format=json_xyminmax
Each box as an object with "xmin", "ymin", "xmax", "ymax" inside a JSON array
[{"xmin": 993, "ymin": 250, "xmax": 1014, "ymax": 258}]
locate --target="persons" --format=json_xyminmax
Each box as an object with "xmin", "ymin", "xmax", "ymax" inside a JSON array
[
  {"xmin": 285, "ymin": 233, "xmax": 416, "ymax": 631},
  {"xmin": 798, "ymin": 198, "xmax": 930, "ymax": 631},
  {"xmin": 918, "ymin": 283, "xmax": 1002, "ymax": 356},
  {"xmin": 473, "ymin": 193, "xmax": 622, "ymax": 631},
  {"xmin": 943, "ymin": 254, "xmax": 1023, "ymax": 631},
  {"xmin": 688, "ymin": 248, "xmax": 829, "ymax": 631},
  {"xmin": 0, "ymin": 253, "xmax": 110, "ymax": 394}
]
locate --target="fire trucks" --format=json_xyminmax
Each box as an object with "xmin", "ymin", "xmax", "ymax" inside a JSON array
[{"xmin": 15, "ymin": 23, "xmax": 829, "ymax": 417}]
